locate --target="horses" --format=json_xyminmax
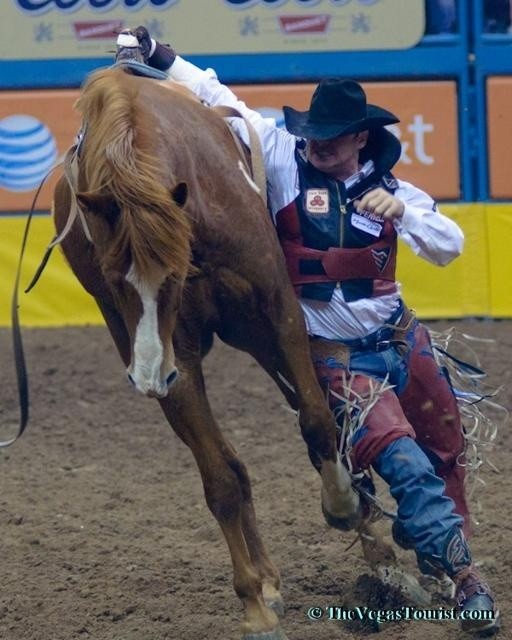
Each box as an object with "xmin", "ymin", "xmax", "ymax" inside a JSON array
[{"xmin": 50, "ymin": 63, "xmax": 433, "ymax": 640}]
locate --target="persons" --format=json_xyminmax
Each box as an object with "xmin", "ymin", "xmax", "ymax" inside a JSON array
[{"xmin": 111, "ymin": 21, "xmax": 502, "ymax": 638}]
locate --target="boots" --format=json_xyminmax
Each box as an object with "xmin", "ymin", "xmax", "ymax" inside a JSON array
[{"xmin": 430, "ymin": 524, "xmax": 501, "ymax": 637}]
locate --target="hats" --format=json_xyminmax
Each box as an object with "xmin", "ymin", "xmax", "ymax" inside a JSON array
[{"xmin": 282, "ymin": 76, "xmax": 400, "ymax": 140}]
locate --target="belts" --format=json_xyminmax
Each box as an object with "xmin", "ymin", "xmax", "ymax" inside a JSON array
[{"xmin": 342, "ymin": 298, "xmax": 406, "ymax": 348}]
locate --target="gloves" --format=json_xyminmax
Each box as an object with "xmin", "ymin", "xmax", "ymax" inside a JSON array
[{"xmin": 115, "ymin": 25, "xmax": 176, "ymax": 71}]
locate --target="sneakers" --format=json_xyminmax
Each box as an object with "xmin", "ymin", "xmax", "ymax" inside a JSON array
[{"xmin": 413, "ymin": 549, "xmax": 456, "ymax": 605}]
